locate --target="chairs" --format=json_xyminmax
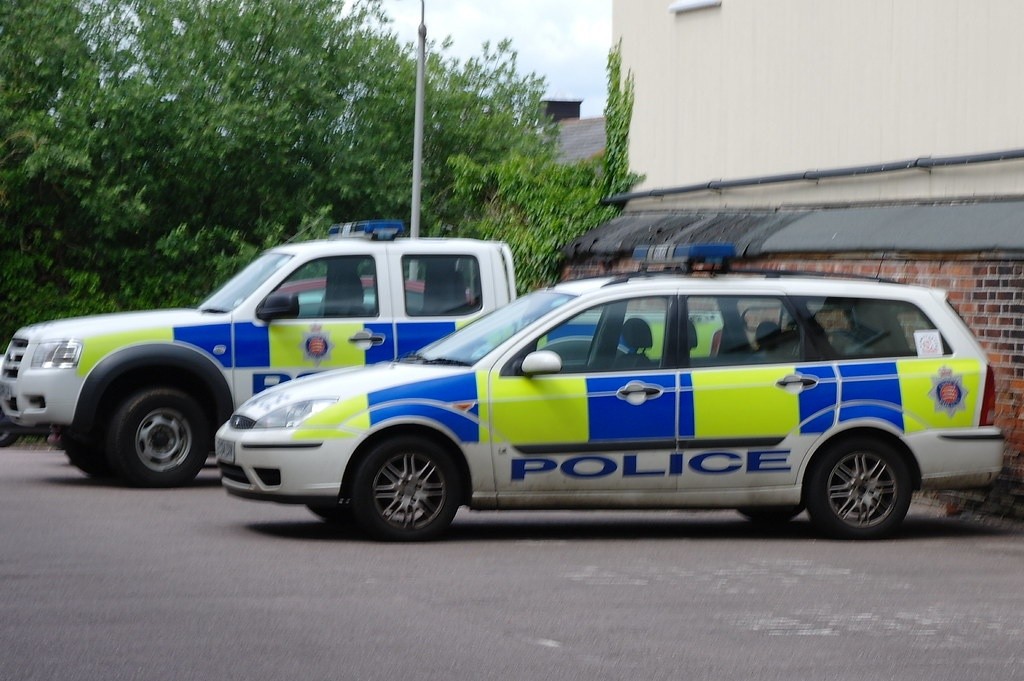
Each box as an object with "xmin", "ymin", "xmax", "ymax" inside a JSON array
[
  {"xmin": 423, "ymin": 271, "xmax": 468, "ymax": 316},
  {"xmin": 739, "ymin": 322, "xmax": 781, "ymax": 364},
  {"xmin": 328, "ymin": 271, "xmax": 366, "ymax": 316},
  {"xmin": 686, "ymin": 320, "xmax": 697, "ymax": 358}
]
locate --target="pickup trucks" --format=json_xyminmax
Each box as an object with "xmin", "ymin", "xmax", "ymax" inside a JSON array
[{"xmin": 0, "ymin": 219, "xmax": 725, "ymax": 490}]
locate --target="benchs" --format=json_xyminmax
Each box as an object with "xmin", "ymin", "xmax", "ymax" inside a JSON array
[{"xmin": 610, "ymin": 318, "xmax": 653, "ymax": 371}]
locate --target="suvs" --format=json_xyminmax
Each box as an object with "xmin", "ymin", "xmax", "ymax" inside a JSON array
[{"xmin": 213, "ymin": 243, "xmax": 1004, "ymax": 547}]
